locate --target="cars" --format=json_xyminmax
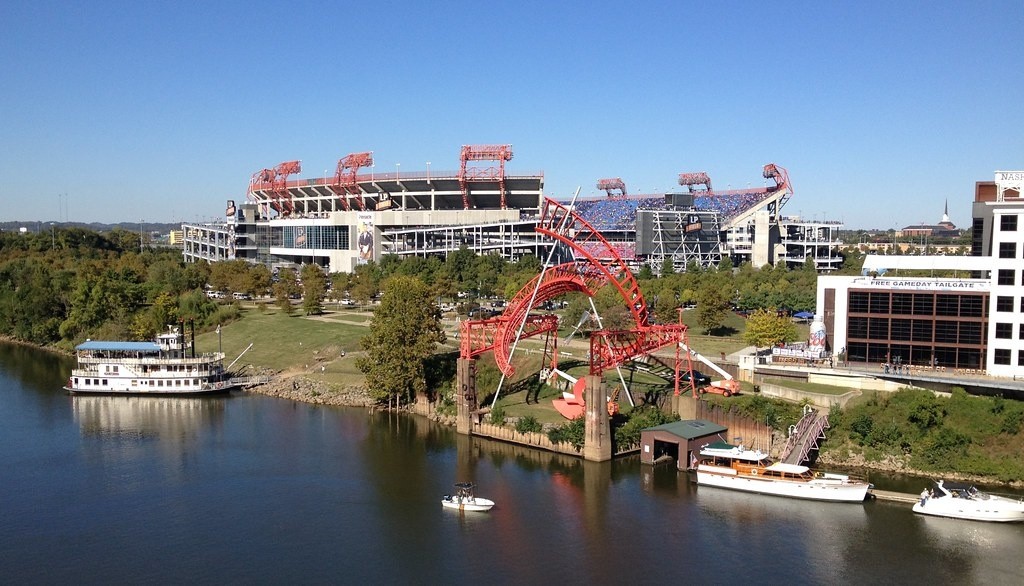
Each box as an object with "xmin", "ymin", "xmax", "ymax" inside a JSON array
[
  {"xmin": 681, "ymin": 370, "xmax": 710, "ymax": 383},
  {"xmin": 440, "ymin": 304, "xmax": 454, "ymax": 312},
  {"xmin": 469, "ymin": 300, "xmax": 504, "ymax": 319},
  {"xmin": 338, "ymin": 298, "xmax": 355, "ymax": 306}
]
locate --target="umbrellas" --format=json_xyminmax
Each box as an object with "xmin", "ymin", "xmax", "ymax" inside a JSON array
[{"xmin": 794, "ymin": 312, "xmax": 814, "ymax": 319}]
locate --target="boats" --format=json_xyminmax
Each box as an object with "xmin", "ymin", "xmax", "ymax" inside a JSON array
[
  {"xmin": 912, "ymin": 479, "xmax": 1024, "ymax": 524},
  {"xmin": 440, "ymin": 481, "xmax": 496, "ymax": 512},
  {"xmin": 61, "ymin": 318, "xmax": 254, "ymax": 398},
  {"xmin": 692, "ymin": 433, "xmax": 874, "ymax": 503}
]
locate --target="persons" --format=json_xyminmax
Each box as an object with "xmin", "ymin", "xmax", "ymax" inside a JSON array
[
  {"xmin": 454, "ymin": 331, "xmax": 458, "ymax": 340},
  {"xmin": 322, "ymin": 365, "xmax": 325, "ymax": 375},
  {"xmin": 341, "ymin": 349, "xmax": 345, "ymax": 357},
  {"xmin": 885, "ymin": 362, "xmax": 909, "ymax": 376},
  {"xmin": 292, "ymin": 380, "xmax": 300, "ymax": 391},
  {"xmin": 358, "ymin": 224, "xmax": 373, "ymax": 259},
  {"xmin": 556, "ymin": 192, "xmax": 766, "ymax": 259},
  {"xmin": 829, "ymin": 357, "xmax": 833, "ymax": 368},
  {"xmin": 920, "ymin": 488, "xmax": 929, "ymax": 507}
]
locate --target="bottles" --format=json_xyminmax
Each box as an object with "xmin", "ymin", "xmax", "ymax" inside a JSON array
[{"xmin": 808, "ymin": 314, "xmax": 826, "ymax": 357}]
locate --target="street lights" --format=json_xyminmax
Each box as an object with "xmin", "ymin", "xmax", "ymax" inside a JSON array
[
  {"xmin": 137, "ymin": 217, "xmax": 145, "ymax": 254},
  {"xmin": 49, "ymin": 222, "xmax": 54, "ymax": 250}
]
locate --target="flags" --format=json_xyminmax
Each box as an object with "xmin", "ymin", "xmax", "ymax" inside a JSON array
[{"xmin": 215, "ymin": 325, "xmax": 220, "ymax": 334}]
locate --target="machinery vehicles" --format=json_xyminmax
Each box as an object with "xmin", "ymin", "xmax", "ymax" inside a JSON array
[{"xmin": 664, "ymin": 330, "xmax": 741, "ymax": 397}]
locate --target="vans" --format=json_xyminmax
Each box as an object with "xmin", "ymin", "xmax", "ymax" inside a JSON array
[{"xmin": 205, "ymin": 290, "xmax": 250, "ymax": 300}]
